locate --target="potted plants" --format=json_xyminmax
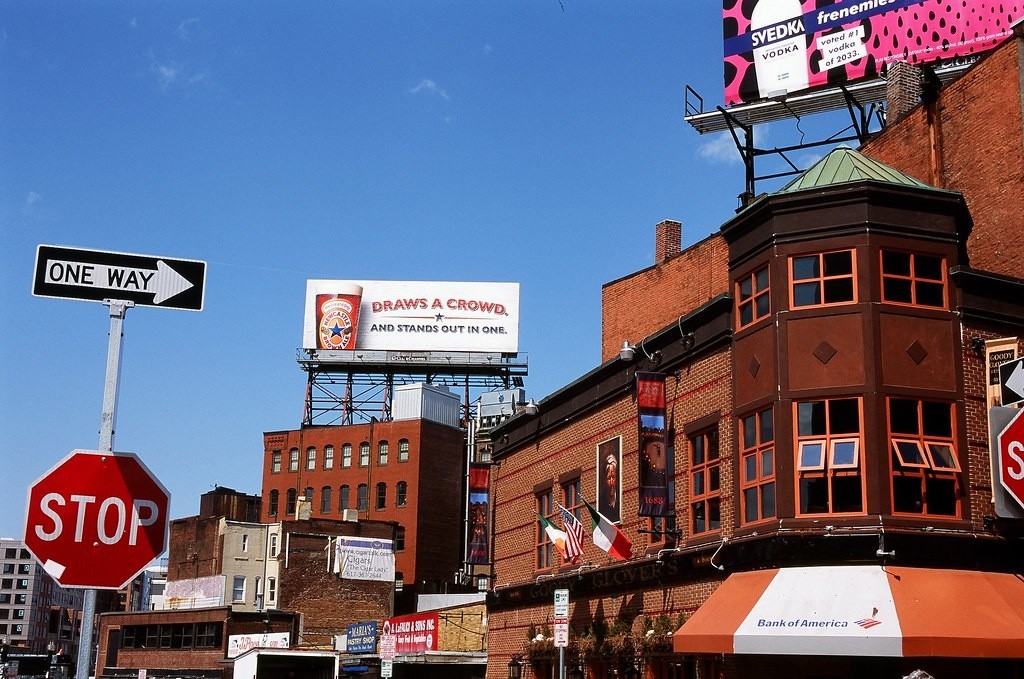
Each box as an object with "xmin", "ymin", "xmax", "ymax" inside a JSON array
[{"xmin": 521, "ymin": 613, "xmax": 686, "ymax": 660}]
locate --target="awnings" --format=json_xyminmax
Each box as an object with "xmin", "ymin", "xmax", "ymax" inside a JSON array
[{"xmin": 673, "ymin": 565, "xmax": 1024, "ymax": 657}]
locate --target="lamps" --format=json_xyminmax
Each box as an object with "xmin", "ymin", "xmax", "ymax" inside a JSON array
[
  {"xmin": 313, "ymin": 354, "xmax": 336, "ymax": 384},
  {"xmin": 768, "ymin": 89, "xmax": 802, "ymax": 122},
  {"xmin": 578, "ymin": 564, "xmax": 601, "ymax": 580},
  {"xmin": 357, "ymin": 355, "xmax": 375, "ymax": 385},
  {"xmin": 656, "ymin": 547, "xmax": 682, "ymax": 565},
  {"xmin": 525, "ymin": 398, "xmax": 537, "ymax": 414},
  {"xmin": 400, "ymin": 355, "xmax": 415, "ymax": 384},
  {"xmin": 710, "ymin": 537, "xmax": 729, "ymax": 571},
  {"xmin": 536, "ymin": 574, "xmax": 557, "ymax": 586},
  {"xmin": 493, "ymin": 583, "xmax": 510, "ymax": 597},
  {"xmin": 487, "ymin": 356, "xmax": 499, "ymax": 387},
  {"xmin": 445, "ymin": 356, "xmax": 458, "ymax": 386},
  {"xmin": 619, "ymin": 339, "xmax": 636, "ymax": 361}
]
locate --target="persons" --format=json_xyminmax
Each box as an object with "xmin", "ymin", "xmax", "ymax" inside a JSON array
[{"xmin": 600, "ymin": 454, "xmax": 620, "ymax": 523}]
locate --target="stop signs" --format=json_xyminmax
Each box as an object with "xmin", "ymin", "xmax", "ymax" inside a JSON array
[
  {"xmin": 997, "ymin": 405, "xmax": 1024, "ymax": 509},
  {"xmin": 22, "ymin": 449, "xmax": 172, "ymax": 589}
]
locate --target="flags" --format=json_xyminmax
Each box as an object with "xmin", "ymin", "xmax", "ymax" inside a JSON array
[
  {"xmin": 580, "ymin": 496, "xmax": 633, "ymax": 560},
  {"xmin": 557, "ymin": 504, "xmax": 585, "ymax": 566},
  {"xmin": 537, "ymin": 513, "xmax": 582, "ymax": 564}
]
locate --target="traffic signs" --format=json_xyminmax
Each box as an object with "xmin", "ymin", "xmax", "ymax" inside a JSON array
[{"xmin": 32, "ymin": 244, "xmax": 208, "ymax": 313}]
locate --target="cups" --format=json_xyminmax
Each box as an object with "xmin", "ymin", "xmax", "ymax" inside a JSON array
[{"xmin": 316, "ymin": 283, "xmax": 362, "ymax": 349}]
